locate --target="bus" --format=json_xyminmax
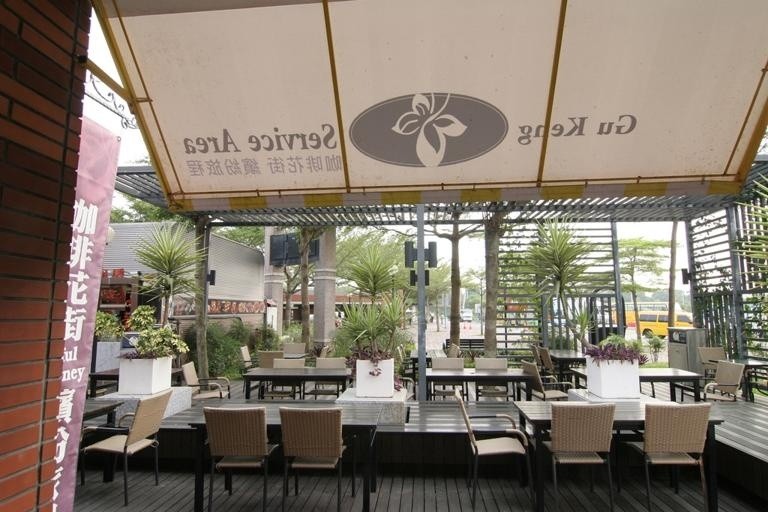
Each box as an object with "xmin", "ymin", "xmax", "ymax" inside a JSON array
[{"xmin": 606, "ymin": 301, "xmax": 685, "ymax": 331}]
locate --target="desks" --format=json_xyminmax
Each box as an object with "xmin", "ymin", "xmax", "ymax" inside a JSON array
[
  {"xmin": 709, "ymin": 358, "xmax": 768, "ymax": 403},
  {"xmin": 78, "ymin": 397, "xmax": 125, "ymax": 486},
  {"xmin": 549, "ymin": 348, "xmax": 588, "ymax": 382},
  {"xmin": 569, "ymin": 367, "xmax": 705, "ymax": 403},
  {"xmin": 187, "ymin": 401, "xmax": 386, "ymax": 512},
  {"xmin": 512, "ymin": 400, "xmax": 728, "ymax": 512}
]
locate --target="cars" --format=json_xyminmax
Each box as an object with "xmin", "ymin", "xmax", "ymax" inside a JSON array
[{"xmin": 404, "ymin": 309, "xmax": 445, "ymax": 324}]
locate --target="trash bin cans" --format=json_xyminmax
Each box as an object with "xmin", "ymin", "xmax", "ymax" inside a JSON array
[{"xmin": 667, "ymin": 327, "xmax": 707, "ymax": 387}]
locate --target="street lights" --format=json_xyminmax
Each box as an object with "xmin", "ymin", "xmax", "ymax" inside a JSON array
[{"xmin": 386, "ymin": 259, "xmax": 401, "ymax": 302}]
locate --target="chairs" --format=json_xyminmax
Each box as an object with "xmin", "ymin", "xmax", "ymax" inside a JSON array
[
  {"xmin": 181, "ymin": 361, "xmax": 231, "ymax": 406},
  {"xmin": 541, "ymin": 404, "xmax": 616, "ymax": 512},
  {"xmin": 201, "ymin": 406, "xmax": 281, "ymax": 512},
  {"xmin": 279, "ymin": 408, "xmax": 357, "ymax": 512},
  {"xmin": 396, "ymin": 343, "xmax": 575, "ymax": 402},
  {"xmin": 622, "ymin": 402, "xmax": 713, "ymax": 512},
  {"xmin": 680, "ymin": 360, "xmax": 746, "ymax": 402},
  {"xmin": 696, "ymin": 347, "xmax": 737, "ymax": 401},
  {"xmin": 239, "ymin": 342, "xmax": 348, "ymax": 401},
  {"xmin": 453, "ymin": 386, "xmax": 537, "ymax": 512},
  {"xmin": 80, "ymin": 388, "xmax": 174, "ymax": 505}
]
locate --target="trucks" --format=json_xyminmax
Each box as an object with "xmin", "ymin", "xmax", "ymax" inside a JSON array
[{"xmin": 458, "ymin": 309, "xmax": 474, "ymax": 323}]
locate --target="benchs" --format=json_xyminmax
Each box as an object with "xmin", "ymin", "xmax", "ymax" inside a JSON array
[{"xmin": 441, "ymin": 337, "xmax": 485, "ymax": 351}]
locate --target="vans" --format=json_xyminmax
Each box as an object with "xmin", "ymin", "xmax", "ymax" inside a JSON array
[{"xmin": 635, "ymin": 310, "xmax": 697, "ymax": 341}]
